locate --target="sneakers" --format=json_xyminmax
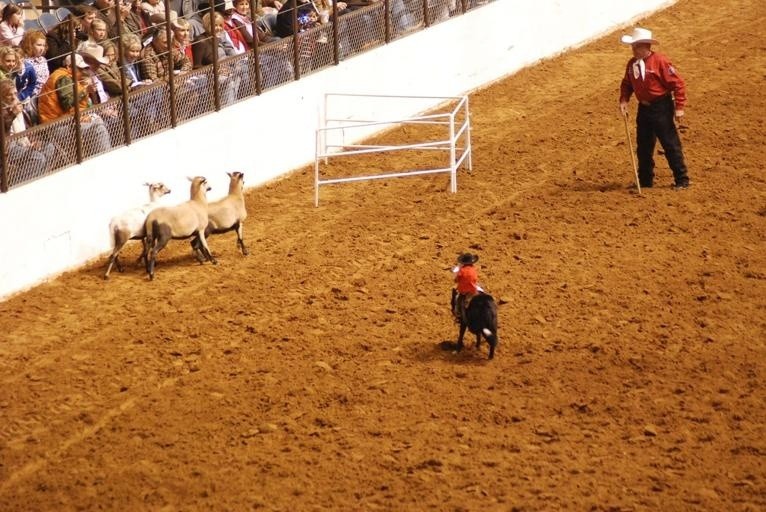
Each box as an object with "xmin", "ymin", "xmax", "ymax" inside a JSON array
[
  {"xmin": 634, "ymin": 182, "xmax": 653, "ymax": 187},
  {"xmin": 675, "ymin": 176, "xmax": 689, "ymax": 189}
]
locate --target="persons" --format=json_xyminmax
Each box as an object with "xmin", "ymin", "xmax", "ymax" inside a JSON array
[
  {"xmin": 0, "ymin": 1, "xmax": 488, "ymax": 192},
  {"xmin": 619, "ymin": 26, "xmax": 690, "ymax": 190},
  {"xmin": 453, "ymin": 253, "xmax": 480, "ymax": 323}
]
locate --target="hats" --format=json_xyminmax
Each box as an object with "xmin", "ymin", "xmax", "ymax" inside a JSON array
[
  {"xmin": 621, "ymin": 27, "xmax": 660, "ymax": 46},
  {"xmin": 80, "ymin": 42, "xmax": 111, "ymax": 66},
  {"xmin": 63, "ymin": 53, "xmax": 89, "ymax": 71},
  {"xmin": 457, "ymin": 252, "xmax": 479, "ymax": 265}
]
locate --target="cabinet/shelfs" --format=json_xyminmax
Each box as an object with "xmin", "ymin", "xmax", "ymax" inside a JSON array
[{"xmin": 315, "ymin": 93, "xmax": 473, "ymax": 208}]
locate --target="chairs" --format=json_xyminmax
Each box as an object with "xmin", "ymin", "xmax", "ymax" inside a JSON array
[
  {"xmin": 37, "ymin": 12, "xmax": 60, "ymax": 35},
  {"xmin": 55, "ymin": 7, "xmax": 72, "ymax": 22},
  {"xmin": 31, "ymin": 94, "xmax": 69, "ymax": 164},
  {"xmin": 260, "ymin": 14, "xmax": 274, "ymax": 36}
]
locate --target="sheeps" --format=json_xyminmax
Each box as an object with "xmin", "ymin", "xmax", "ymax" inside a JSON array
[
  {"xmin": 142, "ymin": 174, "xmax": 220, "ymax": 282},
  {"xmin": 103, "ymin": 180, "xmax": 172, "ymax": 282},
  {"xmin": 449, "ymin": 287, "xmax": 500, "ymax": 361},
  {"xmin": 189, "ymin": 171, "xmax": 250, "ymax": 265}
]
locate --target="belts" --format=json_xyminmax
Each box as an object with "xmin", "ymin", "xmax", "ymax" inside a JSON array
[{"xmin": 641, "ymin": 96, "xmax": 664, "ymax": 106}]
